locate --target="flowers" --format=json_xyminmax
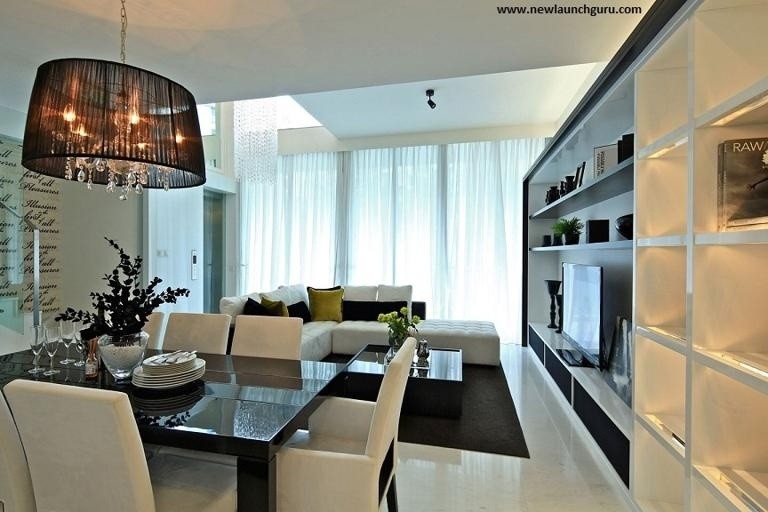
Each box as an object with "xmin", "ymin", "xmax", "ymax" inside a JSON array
[{"xmin": 374, "ymin": 308, "xmax": 421, "ymax": 345}]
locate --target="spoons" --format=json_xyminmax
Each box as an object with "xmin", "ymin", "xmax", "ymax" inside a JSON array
[{"xmin": 167, "ymin": 349, "xmax": 198, "ymax": 363}]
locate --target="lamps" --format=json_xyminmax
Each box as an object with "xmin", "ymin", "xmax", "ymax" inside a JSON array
[
  {"xmin": 424, "ymin": 88, "xmax": 437, "ymax": 110},
  {"xmin": 18, "ymin": 0, "xmax": 208, "ymax": 203}
]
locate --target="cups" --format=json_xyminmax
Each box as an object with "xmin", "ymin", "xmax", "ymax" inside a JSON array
[{"xmin": 541, "ymin": 234, "xmax": 552, "ymax": 247}]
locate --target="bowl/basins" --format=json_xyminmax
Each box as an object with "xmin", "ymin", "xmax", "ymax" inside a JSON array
[{"xmin": 615, "ymin": 213, "xmax": 632, "ymax": 240}]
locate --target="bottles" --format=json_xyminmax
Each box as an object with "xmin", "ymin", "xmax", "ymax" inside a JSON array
[{"xmin": 85, "ymin": 352, "xmax": 99, "ymax": 378}]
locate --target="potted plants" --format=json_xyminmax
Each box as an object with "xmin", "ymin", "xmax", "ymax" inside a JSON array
[
  {"xmin": 52, "ymin": 235, "xmax": 192, "ymax": 382},
  {"xmin": 548, "ymin": 216, "xmax": 585, "ymax": 246}
]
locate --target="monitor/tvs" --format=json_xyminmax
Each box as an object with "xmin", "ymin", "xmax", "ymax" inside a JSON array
[{"xmin": 554, "ymin": 262, "xmax": 607, "ymax": 373}]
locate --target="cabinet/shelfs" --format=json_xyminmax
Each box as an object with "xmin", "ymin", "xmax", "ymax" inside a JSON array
[
  {"xmin": 519, "ymin": 69, "xmax": 634, "ymax": 254},
  {"xmin": 525, "ymin": 319, "xmax": 630, "ymax": 494},
  {"xmin": 630, "ymin": 0, "xmax": 768, "ymax": 512}
]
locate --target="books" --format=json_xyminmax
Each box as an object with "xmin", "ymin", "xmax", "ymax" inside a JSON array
[{"xmin": 717, "ymin": 139, "xmax": 768, "ymax": 228}]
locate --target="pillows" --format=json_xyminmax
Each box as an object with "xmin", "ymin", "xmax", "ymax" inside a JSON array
[{"xmin": 218, "ymin": 282, "xmax": 415, "ymax": 329}]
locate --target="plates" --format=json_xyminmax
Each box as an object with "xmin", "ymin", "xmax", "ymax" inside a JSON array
[
  {"xmin": 129, "ymin": 352, "xmax": 207, "ymax": 388},
  {"xmin": 134, "ymin": 378, "xmax": 205, "ymax": 416}
]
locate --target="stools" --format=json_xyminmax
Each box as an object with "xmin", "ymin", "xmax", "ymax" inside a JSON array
[
  {"xmin": 410, "ymin": 318, "xmax": 503, "ymax": 368},
  {"xmin": 329, "ymin": 319, "xmax": 396, "ymax": 358}
]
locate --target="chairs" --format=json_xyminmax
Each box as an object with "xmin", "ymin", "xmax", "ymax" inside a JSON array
[
  {"xmin": 3, "ymin": 377, "xmax": 240, "ymax": 512},
  {"xmin": 154, "ymin": 308, "xmax": 303, "ymax": 467},
  {"xmin": 258, "ymin": 335, "xmax": 417, "ymax": 510},
  {"xmin": 142, "ymin": 310, "xmax": 233, "ymax": 456},
  {"xmin": 0, "ymin": 387, "xmax": 36, "ymax": 512},
  {"xmin": 134, "ymin": 311, "xmax": 168, "ymax": 351}
]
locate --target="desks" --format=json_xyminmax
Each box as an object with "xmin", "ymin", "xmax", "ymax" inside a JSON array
[{"xmin": 0, "ymin": 341, "xmax": 350, "ymax": 512}]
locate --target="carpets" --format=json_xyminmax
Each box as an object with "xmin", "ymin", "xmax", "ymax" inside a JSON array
[{"xmin": 316, "ymin": 352, "xmax": 530, "ymax": 462}]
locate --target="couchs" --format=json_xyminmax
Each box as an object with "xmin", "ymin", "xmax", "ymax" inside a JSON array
[{"xmin": 215, "ymin": 280, "xmax": 337, "ymax": 363}]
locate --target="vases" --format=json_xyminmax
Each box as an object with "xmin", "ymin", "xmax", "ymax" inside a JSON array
[{"xmin": 381, "ymin": 343, "xmax": 400, "ymax": 368}]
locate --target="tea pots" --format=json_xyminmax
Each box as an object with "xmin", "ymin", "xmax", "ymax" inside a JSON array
[
  {"xmin": 559, "ymin": 176, "xmax": 575, "ymax": 197},
  {"xmin": 416, "ymin": 338, "xmax": 430, "ymax": 359},
  {"xmin": 546, "ymin": 186, "xmax": 561, "ymax": 204}
]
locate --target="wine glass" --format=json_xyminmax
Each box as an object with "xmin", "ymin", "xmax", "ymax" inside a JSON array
[
  {"xmin": 61, "ymin": 320, "xmax": 76, "ymax": 365},
  {"xmin": 43, "ymin": 326, "xmax": 61, "ymax": 376},
  {"xmin": 543, "ymin": 279, "xmax": 563, "ymax": 334},
  {"xmin": 75, "ymin": 332, "xmax": 85, "ymax": 366},
  {"xmin": 27, "ymin": 324, "xmax": 45, "ymax": 373}
]
